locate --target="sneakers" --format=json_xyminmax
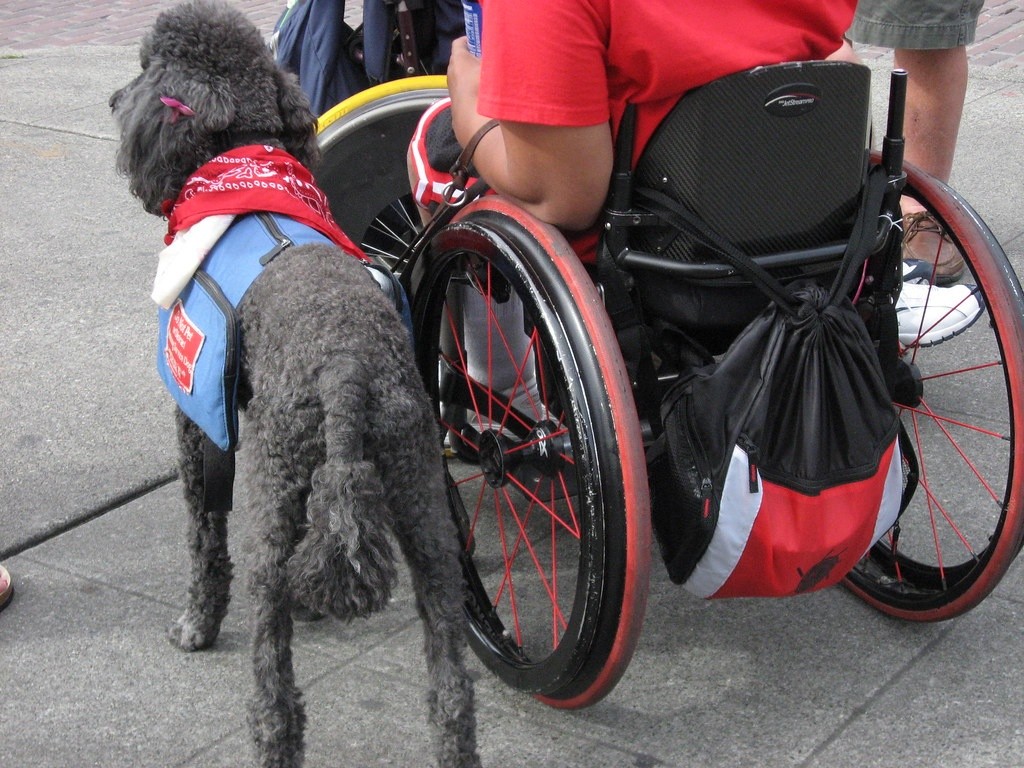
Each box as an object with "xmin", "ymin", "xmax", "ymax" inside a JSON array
[
  {"xmin": 902, "ymin": 258, "xmax": 935, "ymax": 286},
  {"xmin": 894, "ymin": 283, "xmax": 986, "ymax": 358}
]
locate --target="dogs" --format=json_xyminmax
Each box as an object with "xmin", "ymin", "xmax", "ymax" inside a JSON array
[{"xmin": 111, "ymin": 1, "xmax": 474, "ymax": 768}]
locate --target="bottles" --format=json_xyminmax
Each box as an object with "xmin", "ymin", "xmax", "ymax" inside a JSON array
[{"xmin": 461, "ymin": 0, "xmax": 482, "ymax": 60}]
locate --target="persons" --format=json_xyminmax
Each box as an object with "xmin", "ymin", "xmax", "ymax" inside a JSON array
[
  {"xmin": 823, "ymin": 0, "xmax": 986, "ymax": 286},
  {"xmin": 407, "ymin": 0, "xmax": 860, "ymax": 465},
  {"xmin": 891, "ymin": 260, "xmax": 998, "ymax": 350}
]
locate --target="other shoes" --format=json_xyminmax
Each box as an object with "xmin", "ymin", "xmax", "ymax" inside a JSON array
[{"xmin": 901, "ymin": 212, "xmax": 963, "ymax": 283}]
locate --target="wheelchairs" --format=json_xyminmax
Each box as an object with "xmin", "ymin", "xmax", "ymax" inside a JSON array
[{"xmin": 405, "ymin": 60, "xmax": 1023, "ymax": 711}]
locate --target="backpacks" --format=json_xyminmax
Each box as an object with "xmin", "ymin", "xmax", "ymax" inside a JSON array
[{"xmin": 631, "ymin": 169, "xmax": 923, "ymax": 598}]
